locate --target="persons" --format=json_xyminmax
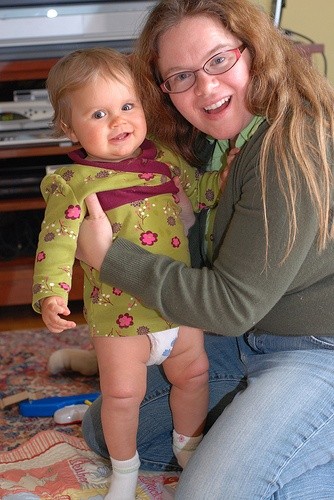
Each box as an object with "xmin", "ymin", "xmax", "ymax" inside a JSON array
[
  {"xmin": 74, "ymin": 0, "xmax": 334, "ymax": 499},
  {"xmin": 31, "ymin": 48, "xmax": 240, "ymax": 500}
]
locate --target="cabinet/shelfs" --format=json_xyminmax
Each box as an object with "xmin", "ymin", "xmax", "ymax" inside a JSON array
[{"xmin": 0, "ymin": 0, "xmax": 327, "ymax": 308}]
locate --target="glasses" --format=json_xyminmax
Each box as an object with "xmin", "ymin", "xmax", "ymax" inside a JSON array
[{"xmin": 160, "ymin": 43, "xmax": 246, "ymax": 93}]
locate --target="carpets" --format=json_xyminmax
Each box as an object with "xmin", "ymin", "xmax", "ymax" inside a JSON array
[{"xmin": 0, "ymin": 324, "xmax": 181, "ymax": 500}]
